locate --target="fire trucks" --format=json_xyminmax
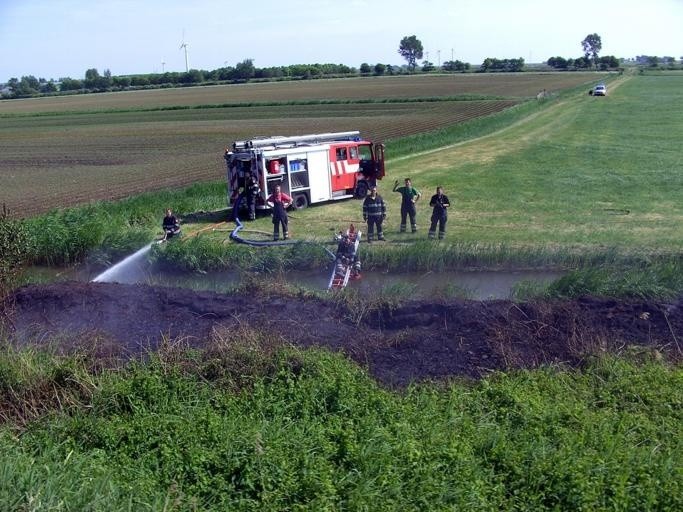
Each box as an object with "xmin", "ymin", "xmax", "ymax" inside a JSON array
[{"xmin": 222, "ymin": 130, "xmax": 385, "ymax": 211}]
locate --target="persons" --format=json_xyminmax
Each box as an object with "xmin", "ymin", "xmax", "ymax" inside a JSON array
[
  {"xmin": 161, "ymin": 207, "xmax": 180, "ymax": 243},
  {"xmin": 246, "ymin": 176, "xmax": 260, "ymax": 222},
  {"xmin": 264, "ymin": 184, "xmax": 294, "ymax": 240},
  {"xmin": 243, "ymin": 170, "xmax": 256, "ymax": 216},
  {"xmin": 391, "ymin": 178, "xmax": 422, "ymax": 235},
  {"xmin": 427, "ymin": 187, "xmax": 449, "ymax": 240},
  {"xmin": 361, "ymin": 186, "xmax": 388, "ymax": 243},
  {"xmin": 346, "ymin": 223, "xmax": 356, "ymax": 247},
  {"xmin": 334, "ymin": 235, "xmax": 361, "ymax": 279}
]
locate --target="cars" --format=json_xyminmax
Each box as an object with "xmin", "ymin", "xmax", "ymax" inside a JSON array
[{"xmin": 593, "ymin": 84, "xmax": 606, "ymax": 96}]
